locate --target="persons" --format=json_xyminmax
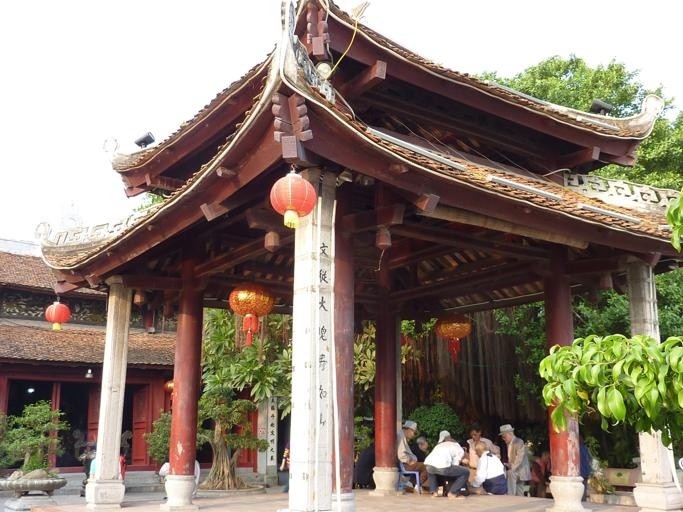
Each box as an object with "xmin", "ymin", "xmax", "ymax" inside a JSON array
[
  {"xmin": 279, "ymin": 439, "xmax": 291, "ymax": 493},
  {"xmin": 120, "ymin": 447, "xmax": 128, "ymax": 505},
  {"xmin": 354, "ymin": 419, "xmax": 592, "ymax": 501}
]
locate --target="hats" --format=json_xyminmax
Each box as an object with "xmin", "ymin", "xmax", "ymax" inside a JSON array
[
  {"xmin": 497, "ymin": 424, "xmax": 515, "ymax": 436},
  {"xmin": 438, "ymin": 430, "xmax": 450, "ymax": 444},
  {"xmin": 402, "ymin": 420, "xmax": 418, "ymax": 433}
]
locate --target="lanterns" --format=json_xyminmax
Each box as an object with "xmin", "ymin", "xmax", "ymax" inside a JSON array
[
  {"xmin": 268, "ymin": 172, "xmax": 317, "ymax": 230},
  {"xmin": 228, "ymin": 281, "xmax": 275, "ymax": 346},
  {"xmin": 161, "ymin": 378, "xmax": 173, "ymax": 400},
  {"xmin": 434, "ymin": 313, "xmax": 472, "ymax": 362},
  {"xmin": 43, "ymin": 301, "xmax": 72, "ymax": 330}
]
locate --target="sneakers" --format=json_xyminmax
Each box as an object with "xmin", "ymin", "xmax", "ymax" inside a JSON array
[
  {"xmin": 432, "ymin": 491, "xmax": 439, "ymax": 496},
  {"xmin": 405, "ymin": 481, "xmax": 428, "ymax": 493},
  {"xmin": 448, "ymin": 492, "xmax": 465, "ymax": 499}
]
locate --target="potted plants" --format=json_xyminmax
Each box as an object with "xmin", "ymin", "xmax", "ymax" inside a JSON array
[{"xmin": 0, "ymin": 400, "xmax": 68, "ymax": 497}]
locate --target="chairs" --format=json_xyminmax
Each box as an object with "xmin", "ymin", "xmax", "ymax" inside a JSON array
[{"xmin": 395, "ymin": 455, "xmax": 422, "ymax": 495}]
